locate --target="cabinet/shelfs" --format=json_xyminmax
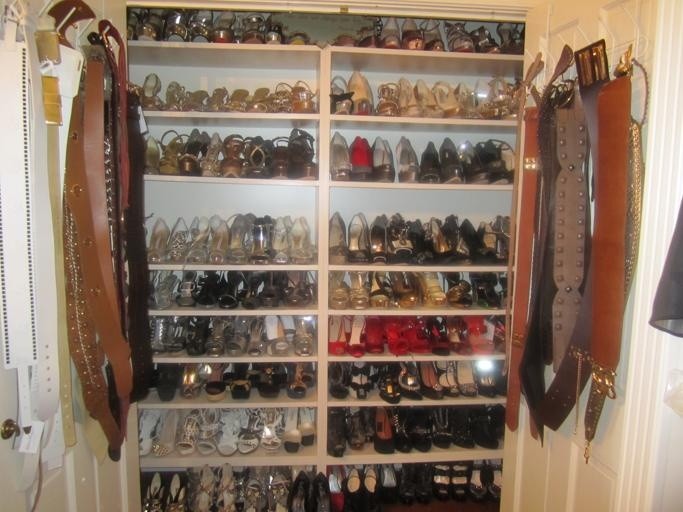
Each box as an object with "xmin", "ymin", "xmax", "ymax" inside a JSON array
[{"xmin": 100, "ymin": 2, "xmax": 535, "ymax": 511}]
